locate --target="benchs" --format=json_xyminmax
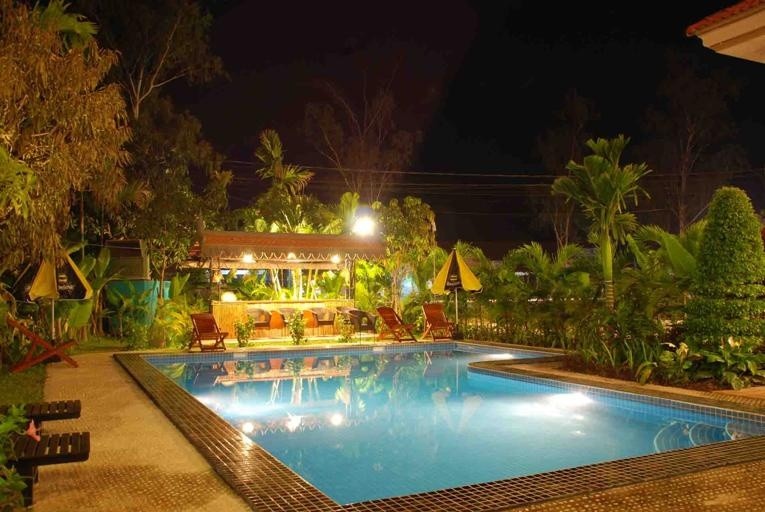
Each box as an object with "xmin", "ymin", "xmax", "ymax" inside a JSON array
[{"xmin": 0, "ymin": 399, "xmax": 93, "ymax": 512}]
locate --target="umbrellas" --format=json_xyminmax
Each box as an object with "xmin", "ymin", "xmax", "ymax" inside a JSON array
[
  {"xmin": 11, "ymin": 238, "xmax": 91, "ymax": 338},
  {"xmin": 429, "ymin": 246, "xmax": 485, "ymax": 326}
]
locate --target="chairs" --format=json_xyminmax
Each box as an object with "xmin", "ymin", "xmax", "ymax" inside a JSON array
[
  {"xmin": 191, "ymin": 361, "xmax": 228, "ymax": 389},
  {"xmin": 245, "ymin": 305, "xmax": 379, "ymax": 345},
  {"xmin": 376, "ymin": 302, "xmax": 456, "ymax": 342},
  {"xmin": 6, "ymin": 316, "xmax": 79, "ymax": 374},
  {"xmin": 376, "ymin": 351, "xmax": 452, "ymax": 378},
  {"xmin": 188, "ymin": 311, "xmax": 229, "ymax": 353}
]
